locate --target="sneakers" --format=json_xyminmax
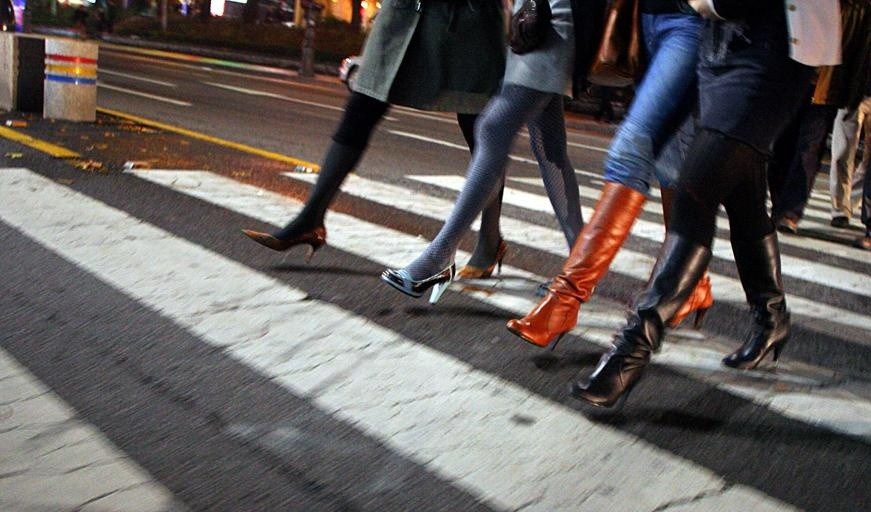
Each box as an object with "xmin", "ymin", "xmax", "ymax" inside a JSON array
[
  {"xmin": 854, "ymin": 235, "xmax": 871, "ymax": 250},
  {"xmin": 831, "ymin": 216, "xmax": 850, "ymax": 228},
  {"xmin": 771, "ymin": 215, "xmax": 798, "ymax": 234}
]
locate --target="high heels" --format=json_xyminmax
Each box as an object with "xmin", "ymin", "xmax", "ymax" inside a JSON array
[
  {"xmin": 456, "ymin": 240, "xmax": 508, "ymax": 283},
  {"xmin": 241, "ymin": 225, "xmax": 327, "ymax": 264},
  {"xmin": 381, "ymin": 261, "xmax": 455, "ymax": 305}
]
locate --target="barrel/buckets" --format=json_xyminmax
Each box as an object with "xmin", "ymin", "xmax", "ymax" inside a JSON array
[{"xmin": 42, "ymin": 37, "xmax": 100, "ymax": 125}]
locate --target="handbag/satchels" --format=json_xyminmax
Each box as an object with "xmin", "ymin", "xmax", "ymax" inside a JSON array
[
  {"xmin": 585, "ymin": 1, "xmax": 641, "ymax": 88},
  {"xmin": 507, "ymin": 1, "xmax": 550, "ymax": 54}
]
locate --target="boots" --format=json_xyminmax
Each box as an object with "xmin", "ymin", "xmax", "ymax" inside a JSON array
[
  {"xmin": 661, "ymin": 183, "xmax": 714, "ymax": 332},
  {"xmin": 722, "ymin": 226, "xmax": 793, "ymax": 369},
  {"xmin": 568, "ymin": 227, "xmax": 710, "ymax": 415},
  {"xmin": 507, "ymin": 181, "xmax": 645, "ymax": 353}
]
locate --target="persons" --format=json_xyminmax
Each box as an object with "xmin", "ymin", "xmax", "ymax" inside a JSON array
[
  {"xmin": 762, "ymin": 0, "xmax": 845, "ymax": 236},
  {"xmin": 829, "ymin": 0, "xmax": 871, "ymax": 229},
  {"xmin": 854, "ymin": 139, "xmax": 871, "ymax": 251},
  {"xmin": 238, "ymin": 0, "xmax": 509, "ymax": 281},
  {"xmin": 380, "ymin": 0, "xmax": 607, "ymax": 308},
  {"xmin": 568, "ymin": 0, "xmax": 794, "ymax": 415},
  {"xmin": 505, "ymin": 0, "xmax": 709, "ymax": 353}
]
